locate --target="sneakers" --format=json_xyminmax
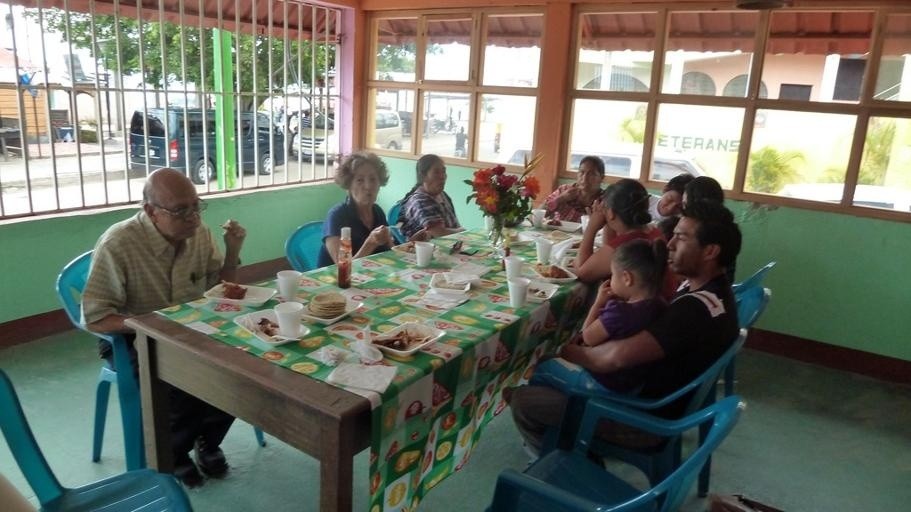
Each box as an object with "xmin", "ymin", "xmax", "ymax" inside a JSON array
[
  {"xmin": 169, "ymin": 454, "xmax": 204, "ymax": 487},
  {"xmin": 193, "ymin": 436, "xmax": 229, "ymax": 475}
]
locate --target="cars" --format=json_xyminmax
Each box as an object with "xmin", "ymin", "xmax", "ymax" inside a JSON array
[{"xmin": 776, "ymin": 182, "xmax": 911, "ymax": 215}]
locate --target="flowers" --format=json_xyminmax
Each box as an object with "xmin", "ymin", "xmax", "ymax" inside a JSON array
[{"xmin": 465, "ymin": 152, "xmax": 546, "ymax": 245}]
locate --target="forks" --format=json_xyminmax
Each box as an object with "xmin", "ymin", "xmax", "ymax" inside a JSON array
[{"xmin": 381, "ymin": 222, "xmax": 404, "ymax": 230}]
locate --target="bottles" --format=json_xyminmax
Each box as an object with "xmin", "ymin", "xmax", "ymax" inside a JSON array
[{"xmin": 338, "ymin": 227, "xmax": 353, "ymax": 288}]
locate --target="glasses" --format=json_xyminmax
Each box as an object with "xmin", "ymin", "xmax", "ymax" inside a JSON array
[{"xmin": 154, "ymin": 197, "xmax": 209, "ymax": 221}]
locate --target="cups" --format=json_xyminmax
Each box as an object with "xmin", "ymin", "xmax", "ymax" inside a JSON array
[
  {"xmin": 580, "ymin": 215, "xmax": 590, "ymax": 234},
  {"xmin": 415, "ymin": 242, "xmax": 434, "ymax": 267},
  {"xmin": 535, "ymin": 239, "xmax": 554, "ymax": 265},
  {"xmin": 503, "ymin": 255, "xmax": 530, "ymax": 308},
  {"xmin": 531, "ymin": 209, "xmax": 546, "ymax": 229}
]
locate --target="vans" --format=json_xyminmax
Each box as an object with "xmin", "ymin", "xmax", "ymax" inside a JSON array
[
  {"xmin": 129, "ymin": 105, "xmax": 286, "ymax": 185},
  {"xmin": 504, "ymin": 137, "xmax": 705, "ymax": 186},
  {"xmin": 295, "ymin": 108, "xmax": 404, "ymax": 161}
]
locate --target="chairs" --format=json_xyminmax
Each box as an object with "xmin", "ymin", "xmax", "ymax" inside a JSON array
[
  {"xmin": 285, "ymin": 220, "xmax": 328, "ymax": 273},
  {"xmin": 486, "ymin": 261, "xmax": 775, "ymax": 512},
  {"xmin": 55, "ymin": 249, "xmax": 268, "ymax": 472},
  {"xmin": 388, "ymin": 199, "xmax": 410, "ymax": 244},
  {"xmin": 0, "ymin": 367, "xmax": 194, "ymax": 511}
]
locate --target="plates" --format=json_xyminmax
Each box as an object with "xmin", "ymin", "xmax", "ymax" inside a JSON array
[
  {"xmin": 429, "ymin": 272, "xmax": 470, "ymax": 295},
  {"xmin": 202, "ymin": 270, "xmax": 365, "ymax": 348},
  {"xmin": 530, "ymin": 265, "xmax": 579, "ymax": 283},
  {"xmin": 368, "ymin": 321, "xmax": 448, "ymax": 359},
  {"xmin": 528, "ymin": 283, "xmax": 561, "ymax": 304},
  {"xmin": 390, "ymin": 241, "xmax": 440, "ymax": 258},
  {"xmin": 543, "ymin": 220, "xmax": 583, "ymax": 234}
]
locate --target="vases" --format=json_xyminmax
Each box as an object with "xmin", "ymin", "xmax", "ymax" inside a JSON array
[{"xmin": 486, "ymin": 217, "xmax": 506, "ymax": 250}]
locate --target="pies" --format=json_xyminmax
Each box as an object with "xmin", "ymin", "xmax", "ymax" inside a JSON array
[{"xmin": 307, "ymin": 292, "xmax": 347, "ymax": 321}]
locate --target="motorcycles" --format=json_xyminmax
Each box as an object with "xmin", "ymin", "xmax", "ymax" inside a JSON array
[
  {"xmin": 432, "ymin": 116, "xmax": 458, "ymax": 136},
  {"xmin": 454, "ymin": 146, "xmax": 468, "ymax": 159}
]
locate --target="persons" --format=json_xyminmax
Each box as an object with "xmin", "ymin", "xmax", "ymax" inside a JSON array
[
  {"xmin": 81, "ymin": 168, "xmax": 247, "ymax": 488},
  {"xmin": 317, "ymin": 150, "xmax": 428, "ymax": 269},
  {"xmin": 399, "ymin": 155, "xmax": 466, "ymax": 237}
]
